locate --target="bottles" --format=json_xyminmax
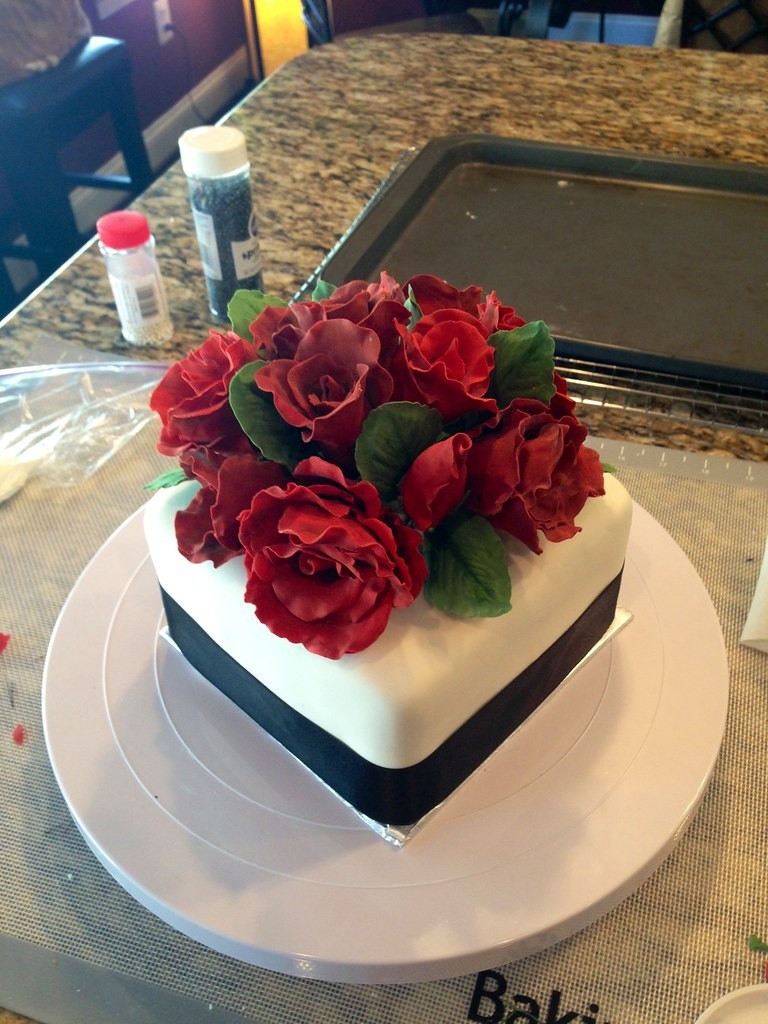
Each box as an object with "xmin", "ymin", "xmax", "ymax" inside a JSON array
[
  {"xmin": 96, "ymin": 210, "xmax": 175, "ymax": 347},
  {"xmin": 176, "ymin": 126, "xmax": 264, "ymax": 323}
]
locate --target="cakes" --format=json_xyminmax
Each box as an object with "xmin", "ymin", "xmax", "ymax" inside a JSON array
[{"xmin": 140, "ymin": 272, "xmax": 633, "ymax": 847}]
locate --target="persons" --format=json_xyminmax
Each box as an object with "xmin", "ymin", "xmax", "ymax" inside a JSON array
[{"xmin": 0, "ymin": 0, "xmax": 95, "ymax": 93}]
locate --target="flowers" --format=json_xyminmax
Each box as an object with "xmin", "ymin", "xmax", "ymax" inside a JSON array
[{"xmin": 150, "ymin": 269, "xmax": 607, "ymax": 660}]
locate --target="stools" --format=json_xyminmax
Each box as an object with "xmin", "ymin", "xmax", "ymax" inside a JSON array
[{"xmin": 0, "ymin": 37, "xmax": 154, "ymax": 323}]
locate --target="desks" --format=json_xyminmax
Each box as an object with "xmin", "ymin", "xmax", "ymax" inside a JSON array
[{"xmin": 0, "ymin": 32, "xmax": 768, "ymax": 1024}]
